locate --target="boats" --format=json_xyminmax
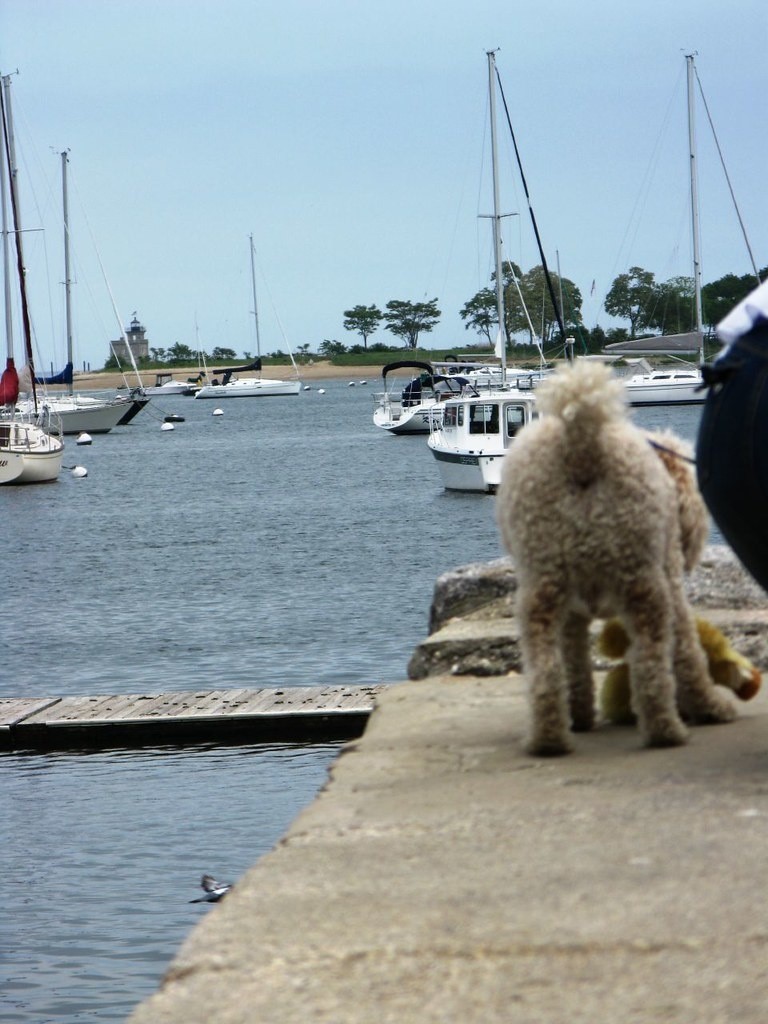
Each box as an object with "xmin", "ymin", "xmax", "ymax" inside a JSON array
[
  {"xmin": 193, "ymin": 236, "xmax": 300, "ymax": 398},
  {"xmin": 428, "ymin": 47, "xmax": 577, "ymax": 494},
  {"xmin": 374, "ymin": 353, "xmax": 511, "ymax": 435},
  {"xmin": 0, "ymin": 68, "xmax": 153, "ymax": 485},
  {"xmin": 132, "ymin": 370, "xmax": 198, "ymax": 397},
  {"xmin": 615, "ymin": 47, "xmax": 762, "ymax": 407}
]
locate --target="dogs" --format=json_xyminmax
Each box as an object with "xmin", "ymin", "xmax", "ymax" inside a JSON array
[{"xmin": 495, "ymin": 356, "xmax": 742, "ymax": 756}]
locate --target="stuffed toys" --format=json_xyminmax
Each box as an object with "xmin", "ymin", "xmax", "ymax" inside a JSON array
[{"xmin": 599, "ymin": 610, "xmax": 764, "ymax": 727}]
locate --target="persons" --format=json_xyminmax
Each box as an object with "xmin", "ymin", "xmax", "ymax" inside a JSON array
[{"xmin": 693, "ymin": 273, "xmax": 768, "ymax": 600}]
